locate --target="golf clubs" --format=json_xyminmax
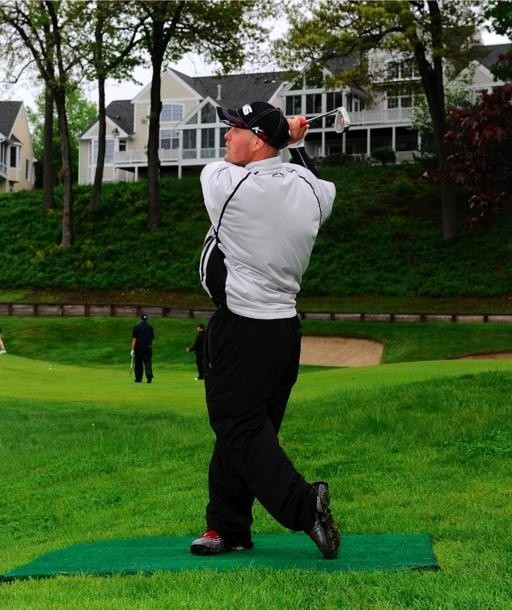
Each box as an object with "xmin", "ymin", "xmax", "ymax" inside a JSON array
[{"xmin": 302, "ymin": 107, "xmax": 351, "ymax": 134}]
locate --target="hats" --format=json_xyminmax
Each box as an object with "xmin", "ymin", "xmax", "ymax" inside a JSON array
[
  {"xmin": 141, "ymin": 314, "xmax": 148, "ymax": 321},
  {"xmin": 216, "ymin": 101, "xmax": 291, "ymax": 150}
]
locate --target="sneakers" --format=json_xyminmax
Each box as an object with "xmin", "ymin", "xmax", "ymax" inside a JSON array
[
  {"xmin": 191, "ymin": 528, "xmax": 253, "ymax": 554},
  {"xmin": 309, "ymin": 481, "xmax": 340, "ymax": 559}
]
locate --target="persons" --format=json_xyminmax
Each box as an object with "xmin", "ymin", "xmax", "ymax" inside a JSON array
[
  {"xmin": 186, "ymin": 325, "xmax": 206, "ymax": 380},
  {"xmin": 0, "ymin": 328, "xmax": 7, "ymax": 354},
  {"xmin": 190, "ymin": 101, "xmax": 340, "ymax": 560},
  {"xmin": 130, "ymin": 314, "xmax": 155, "ymax": 383}
]
